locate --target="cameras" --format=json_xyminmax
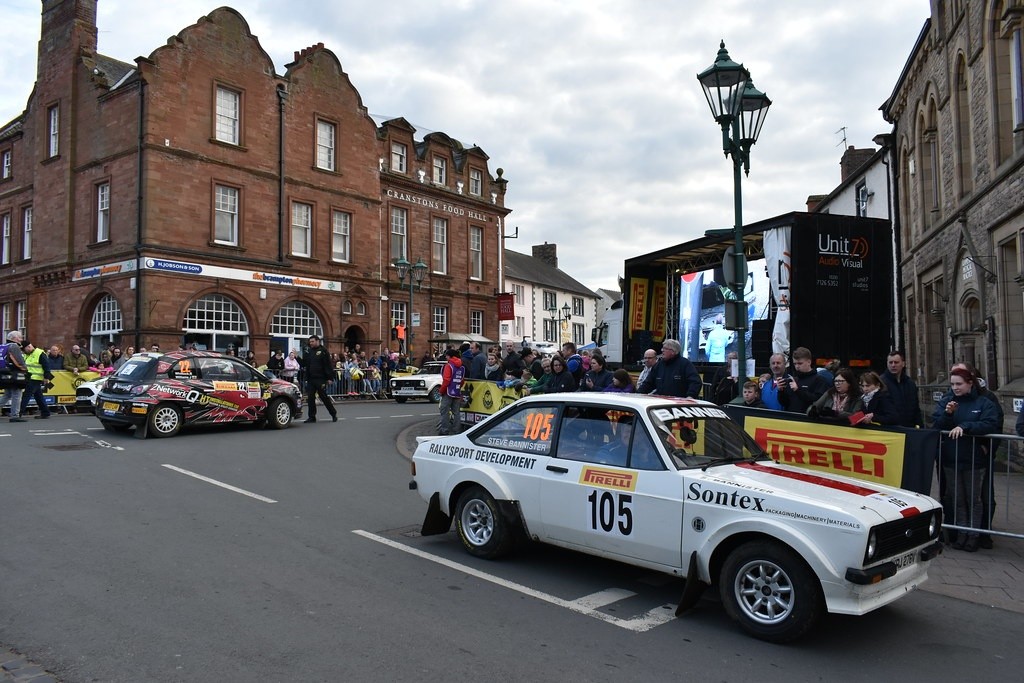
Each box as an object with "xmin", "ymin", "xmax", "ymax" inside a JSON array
[
  {"xmin": 780, "ymin": 378, "xmax": 792, "ymax": 385},
  {"xmin": 507, "ymin": 367, "xmax": 524, "ymax": 378}
]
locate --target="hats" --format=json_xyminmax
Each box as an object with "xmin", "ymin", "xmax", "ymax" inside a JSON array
[{"xmin": 21, "ymin": 341, "xmax": 30, "ymax": 350}]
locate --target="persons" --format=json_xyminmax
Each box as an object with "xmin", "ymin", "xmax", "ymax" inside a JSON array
[
  {"xmin": 596, "ymin": 420, "xmax": 653, "ymax": 470},
  {"xmin": 1015, "ymin": 397, "xmax": 1024, "ymax": 444},
  {"xmin": 279, "ymin": 352, "xmax": 301, "ymax": 383},
  {"xmin": 422, "ymin": 338, "xmax": 703, "ymax": 399},
  {"xmin": 711, "ymin": 347, "xmax": 925, "ymax": 431},
  {"xmin": 395, "ymin": 320, "xmax": 409, "ymax": 357},
  {"xmin": 927, "ymin": 361, "xmax": 1004, "ymax": 553},
  {"xmin": 177, "ymin": 345, "xmax": 188, "ymax": 351},
  {"xmin": 226, "ymin": 348, "xmax": 235, "ymax": 357},
  {"xmin": 244, "ymin": 350, "xmax": 259, "ymax": 369},
  {"xmin": 0, "ymin": 341, "xmax": 134, "ymax": 416},
  {"xmin": 438, "ymin": 348, "xmax": 466, "ymax": 436},
  {"xmin": 326, "ymin": 343, "xmax": 417, "ymax": 402},
  {"xmin": 17, "ymin": 341, "xmax": 52, "ymax": 420},
  {"xmin": 150, "ymin": 343, "xmax": 161, "ymax": 352},
  {"xmin": 139, "ymin": 346, "xmax": 148, "ymax": 353},
  {"xmin": 188, "ymin": 346, "xmax": 199, "ymax": 351},
  {"xmin": 292, "ymin": 335, "xmax": 338, "ymax": 424},
  {"xmin": 0, "ymin": 331, "xmax": 31, "ymax": 422},
  {"xmin": 265, "ymin": 349, "xmax": 285, "ymax": 379}
]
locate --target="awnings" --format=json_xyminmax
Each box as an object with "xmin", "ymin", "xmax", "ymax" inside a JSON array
[{"xmin": 427, "ymin": 332, "xmax": 500, "ymax": 346}]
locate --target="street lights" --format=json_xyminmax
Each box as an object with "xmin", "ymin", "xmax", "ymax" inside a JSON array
[
  {"xmin": 548, "ymin": 302, "xmax": 572, "ymax": 351},
  {"xmin": 696, "ymin": 39, "xmax": 773, "ymax": 399},
  {"xmin": 394, "ymin": 254, "xmax": 428, "ymax": 367}
]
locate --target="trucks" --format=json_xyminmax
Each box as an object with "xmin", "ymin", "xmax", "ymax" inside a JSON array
[{"xmin": 590, "ymin": 211, "xmax": 892, "ymax": 396}]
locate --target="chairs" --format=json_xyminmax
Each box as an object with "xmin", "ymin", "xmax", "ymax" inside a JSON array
[{"xmin": 578, "ymin": 410, "xmax": 615, "ymax": 462}]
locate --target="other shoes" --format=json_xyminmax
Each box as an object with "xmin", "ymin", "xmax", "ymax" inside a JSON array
[
  {"xmin": 294, "ymin": 414, "xmax": 302, "ymax": 419},
  {"xmin": 304, "ymin": 418, "xmax": 316, "ymax": 423},
  {"xmin": 332, "ymin": 413, "xmax": 337, "ymax": 422},
  {"xmin": 9, "ymin": 417, "xmax": 27, "ymax": 422},
  {"xmin": 938, "ymin": 526, "xmax": 994, "ymax": 553},
  {"xmin": 34, "ymin": 415, "xmax": 48, "ymax": 419}
]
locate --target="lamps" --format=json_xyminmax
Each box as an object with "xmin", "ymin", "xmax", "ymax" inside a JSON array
[
  {"xmin": 860, "ymin": 188, "xmax": 874, "ymax": 202},
  {"xmin": 93, "ymin": 68, "xmax": 105, "ymax": 78}
]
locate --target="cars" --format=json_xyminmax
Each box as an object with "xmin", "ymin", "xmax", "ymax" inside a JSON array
[
  {"xmin": 408, "ymin": 392, "xmax": 946, "ymax": 646},
  {"xmin": 73, "ymin": 350, "xmax": 303, "ymax": 440},
  {"xmin": 389, "ymin": 361, "xmax": 449, "ymax": 404}
]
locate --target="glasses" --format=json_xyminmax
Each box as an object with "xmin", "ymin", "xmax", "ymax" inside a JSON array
[
  {"xmin": 73, "ymin": 349, "xmax": 81, "ymax": 351},
  {"xmin": 663, "ymin": 347, "xmax": 673, "ymax": 351},
  {"xmin": 644, "ymin": 357, "xmax": 656, "ymax": 359},
  {"xmin": 834, "ymin": 379, "xmax": 847, "ymax": 385}
]
